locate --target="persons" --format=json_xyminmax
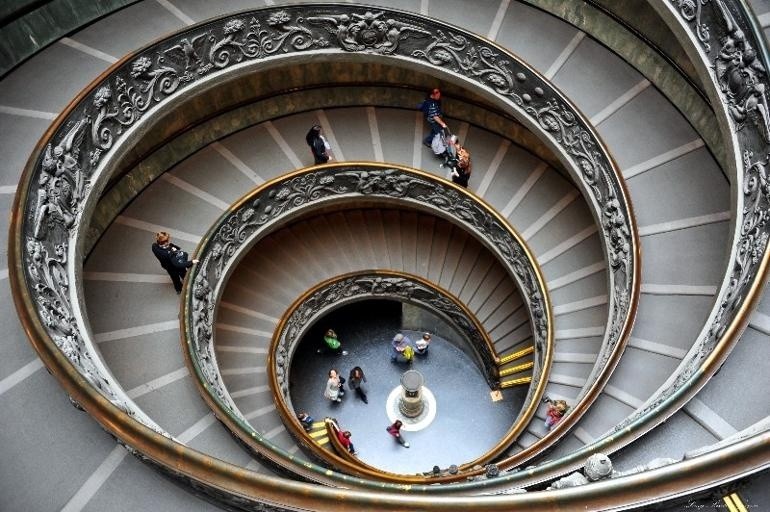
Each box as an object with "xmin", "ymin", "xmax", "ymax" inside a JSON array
[
  {"xmin": 450, "ymin": 147, "xmax": 473, "ymax": 188},
  {"xmin": 152, "ymin": 231, "xmax": 200, "ymax": 292},
  {"xmin": 324, "ymin": 329, "xmax": 349, "ymax": 356},
  {"xmin": 412, "ymin": 332, "xmax": 432, "ymax": 360},
  {"xmin": 325, "ymin": 418, "xmax": 358, "ymax": 455},
  {"xmin": 306, "ymin": 125, "xmax": 334, "ymax": 165},
  {"xmin": 390, "ymin": 333, "xmax": 414, "ymax": 370},
  {"xmin": 348, "ymin": 367, "xmax": 369, "ymax": 404},
  {"xmin": 386, "ymin": 419, "xmax": 411, "ymax": 448},
  {"xmin": 421, "ymin": 89, "xmax": 447, "ymax": 151},
  {"xmin": 298, "ymin": 411, "xmax": 315, "ymax": 433},
  {"xmin": 544, "ymin": 399, "xmax": 569, "ymax": 430},
  {"xmin": 324, "ymin": 368, "xmax": 346, "ymax": 403}
]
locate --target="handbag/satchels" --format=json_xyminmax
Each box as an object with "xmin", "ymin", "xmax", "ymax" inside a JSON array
[
  {"xmin": 170, "ymin": 250, "xmax": 188, "ymax": 264},
  {"xmin": 431, "ymin": 128, "xmax": 446, "ymax": 154},
  {"xmin": 320, "ymin": 134, "xmax": 330, "ymax": 150},
  {"xmin": 323, "ymin": 387, "xmax": 338, "ymax": 401}
]
